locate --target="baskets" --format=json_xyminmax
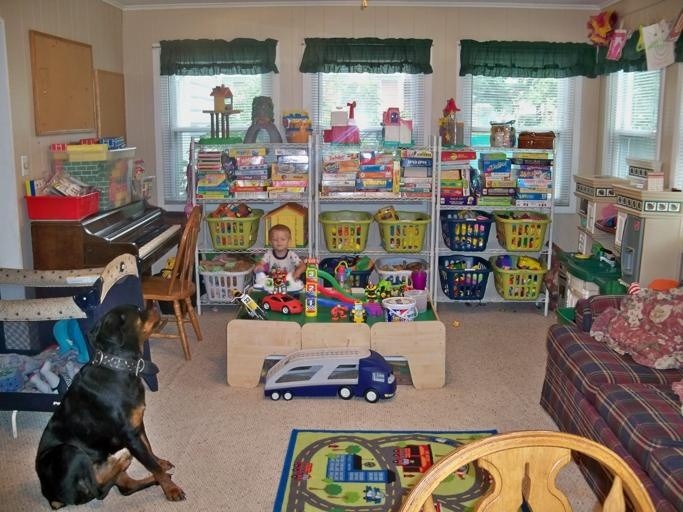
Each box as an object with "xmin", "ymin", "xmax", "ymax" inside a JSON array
[
  {"xmin": 319, "ymin": 258, "xmax": 375, "ymax": 288},
  {"xmin": 493, "ymin": 209, "xmax": 551, "ymax": 252},
  {"xmin": 374, "ymin": 209, "xmax": 432, "ymax": 253},
  {"xmin": 207, "ymin": 209, "xmax": 263, "ymax": 250},
  {"xmin": 374, "ymin": 256, "xmax": 429, "ymax": 290},
  {"xmin": 200, "ymin": 264, "xmax": 256, "ymax": 303},
  {"xmin": 318, "ymin": 211, "xmax": 372, "ymax": 252},
  {"xmin": 440, "ymin": 211, "xmax": 495, "ymax": 252},
  {"xmin": 490, "ymin": 255, "xmax": 548, "ymax": 302}
]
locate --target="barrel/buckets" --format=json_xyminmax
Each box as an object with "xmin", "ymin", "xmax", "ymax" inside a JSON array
[{"xmin": 382, "ymin": 296, "xmax": 419, "ymax": 322}]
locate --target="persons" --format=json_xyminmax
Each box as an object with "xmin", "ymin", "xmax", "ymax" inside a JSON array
[{"xmin": 253, "ymin": 224, "xmax": 306, "ymax": 280}]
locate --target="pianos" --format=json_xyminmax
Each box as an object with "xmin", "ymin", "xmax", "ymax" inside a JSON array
[{"xmin": 30, "ymin": 199, "xmax": 186, "ymax": 308}]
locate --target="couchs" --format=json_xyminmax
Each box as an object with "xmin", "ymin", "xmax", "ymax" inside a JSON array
[{"xmin": 537, "ymin": 292, "xmax": 683, "ymax": 512}]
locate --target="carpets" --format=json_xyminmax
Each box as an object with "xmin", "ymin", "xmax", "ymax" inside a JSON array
[{"xmin": 270, "ymin": 428, "xmax": 533, "ymax": 512}]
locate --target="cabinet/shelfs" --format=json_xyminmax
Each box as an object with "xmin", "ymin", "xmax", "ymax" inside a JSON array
[
  {"xmin": 434, "ymin": 135, "xmax": 557, "ymax": 312},
  {"xmin": 189, "ymin": 135, "xmax": 313, "ymax": 317},
  {"xmin": 312, "ymin": 134, "xmax": 437, "ymax": 302},
  {"xmin": 573, "ymin": 158, "xmax": 683, "ymax": 325}
]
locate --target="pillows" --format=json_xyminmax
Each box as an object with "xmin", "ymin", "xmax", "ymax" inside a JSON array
[
  {"xmin": 672, "ymin": 379, "xmax": 683, "ymax": 403},
  {"xmin": 586, "ymin": 286, "xmax": 683, "ymax": 371}
]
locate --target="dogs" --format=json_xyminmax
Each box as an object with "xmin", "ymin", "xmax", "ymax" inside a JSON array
[{"xmin": 35, "ymin": 303, "xmax": 186, "ymax": 511}]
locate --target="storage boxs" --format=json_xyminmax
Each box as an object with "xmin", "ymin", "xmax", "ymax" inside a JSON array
[
  {"xmin": 23, "ymin": 190, "xmax": 101, "ymax": 220},
  {"xmin": 518, "ymin": 131, "xmax": 556, "ymax": 149},
  {"xmin": 46, "ymin": 145, "xmax": 139, "ymax": 213}
]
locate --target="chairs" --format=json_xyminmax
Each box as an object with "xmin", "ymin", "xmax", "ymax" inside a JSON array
[
  {"xmin": 399, "ymin": 428, "xmax": 657, "ymax": 512},
  {"xmin": 140, "ymin": 205, "xmax": 205, "ymax": 362}
]
